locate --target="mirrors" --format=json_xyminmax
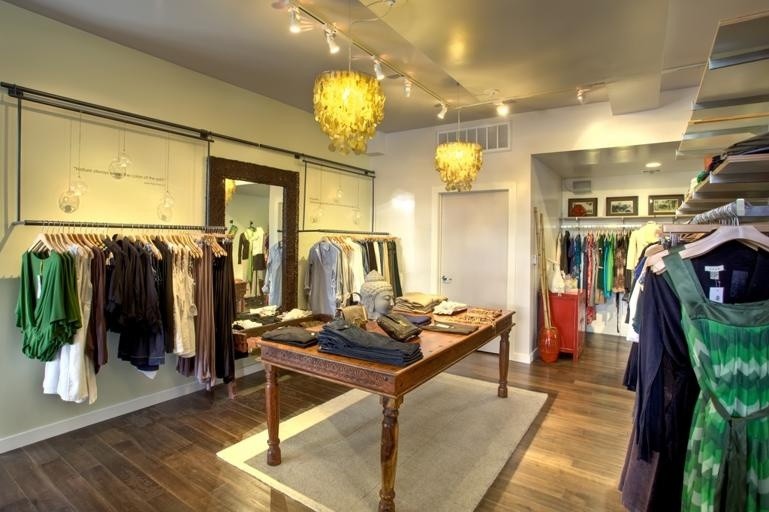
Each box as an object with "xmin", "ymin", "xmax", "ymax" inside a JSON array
[{"xmin": 209, "ymin": 156, "xmax": 298, "ymax": 330}]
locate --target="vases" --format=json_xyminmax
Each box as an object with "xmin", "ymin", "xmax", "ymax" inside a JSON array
[{"xmin": 537, "ymin": 327, "xmax": 560, "ymax": 364}]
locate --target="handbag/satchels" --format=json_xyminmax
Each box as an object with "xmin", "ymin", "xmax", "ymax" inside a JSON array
[
  {"xmin": 376, "ymin": 313, "xmax": 423, "ymax": 343},
  {"xmin": 336, "ymin": 292, "xmax": 369, "ymax": 330}
]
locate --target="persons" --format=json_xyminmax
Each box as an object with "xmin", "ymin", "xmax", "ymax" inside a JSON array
[{"xmin": 361, "ymin": 270, "xmax": 395, "ymax": 320}]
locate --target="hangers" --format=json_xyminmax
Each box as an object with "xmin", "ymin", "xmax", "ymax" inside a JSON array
[
  {"xmin": 646, "ymin": 200, "xmax": 769, "ymax": 277},
  {"xmin": 320, "ymin": 229, "xmax": 396, "ymax": 253},
  {"xmin": 26, "ymin": 219, "xmax": 230, "ymax": 260}
]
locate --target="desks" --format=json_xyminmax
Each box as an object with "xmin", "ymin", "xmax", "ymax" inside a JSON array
[{"xmin": 255, "ymin": 301, "xmax": 516, "ymax": 512}]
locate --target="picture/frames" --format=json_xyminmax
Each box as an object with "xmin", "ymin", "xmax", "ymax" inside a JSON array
[{"xmin": 568, "ymin": 194, "xmax": 684, "ymax": 217}]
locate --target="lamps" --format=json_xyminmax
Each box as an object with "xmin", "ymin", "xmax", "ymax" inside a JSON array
[
  {"xmin": 311, "ymin": 0, "xmax": 388, "ymax": 156},
  {"xmin": 434, "ymin": 83, "xmax": 484, "ymax": 194}
]
